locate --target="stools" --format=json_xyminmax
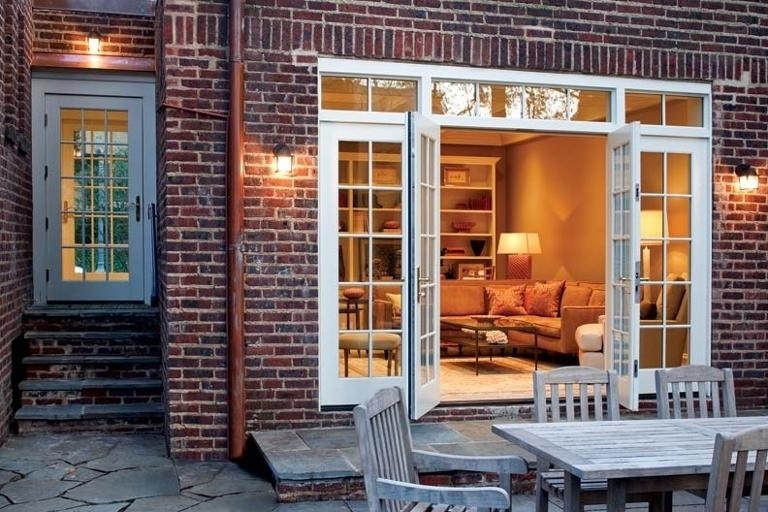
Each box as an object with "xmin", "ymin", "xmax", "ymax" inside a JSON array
[{"xmin": 338, "ymin": 331, "xmax": 401, "ymax": 376}]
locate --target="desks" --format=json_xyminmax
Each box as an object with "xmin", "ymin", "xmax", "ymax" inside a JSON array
[{"xmin": 491, "ymin": 415, "xmax": 768, "ymax": 512}]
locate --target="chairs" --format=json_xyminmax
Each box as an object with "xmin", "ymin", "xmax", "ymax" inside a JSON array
[
  {"xmin": 532, "ymin": 364, "xmax": 768, "ymax": 512},
  {"xmin": 352, "ymin": 386, "xmax": 529, "ymax": 512},
  {"xmin": 574, "ymin": 271, "xmax": 691, "ymax": 369}
]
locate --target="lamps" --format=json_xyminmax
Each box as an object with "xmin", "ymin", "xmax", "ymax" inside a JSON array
[
  {"xmin": 87, "ymin": 29, "xmax": 101, "ymax": 53},
  {"xmin": 736, "ymin": 163, "xmax": 759, "ymax": 190},
  {"xmin": 497, "ymin": 232, "xmax": 543, "ymax": 279},
  {"xmin": 639, "ymin": 209, "xmax": 671, "ymax": 303},
  {"xmin": 272, "ymin": 142, "xmax": 294, "ymax": 174}
]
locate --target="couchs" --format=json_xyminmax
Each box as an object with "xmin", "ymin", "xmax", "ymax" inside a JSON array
[{"xmin": 365, "ymin": 278, "xmax": 644, "ymax": 360}]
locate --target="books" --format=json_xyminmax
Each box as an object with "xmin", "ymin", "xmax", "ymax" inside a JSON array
[
  {"xmin": 380, "ymin": 228, "xmax": 401, "ymax": 233},
  {"xmin": 353, "ymin": 211, "xmax": 368, "ymax": 233}
]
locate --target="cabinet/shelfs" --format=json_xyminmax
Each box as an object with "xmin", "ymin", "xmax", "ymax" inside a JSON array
[{"xmin": 338, "ymin": 150, "xmax": 503, "ymax": 287}]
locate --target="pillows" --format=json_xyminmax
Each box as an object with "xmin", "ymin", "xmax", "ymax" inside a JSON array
[
  {"xmin": 639, "ymin": 299, "xmax": 657, "ymax": 320},
  {"xmin": 482, "ymin": 279, "xmax": 566, "ymax": 318},
  {"xmin": 384, "ymin": 292, "xmax": 402, "ymax": 316}
]
